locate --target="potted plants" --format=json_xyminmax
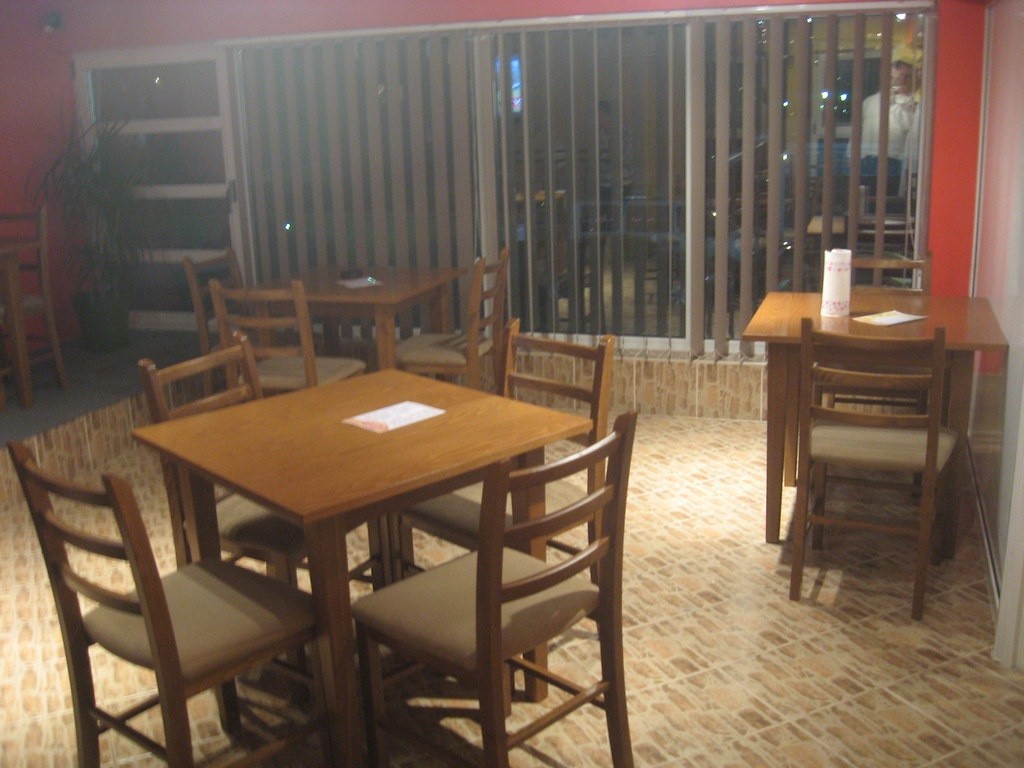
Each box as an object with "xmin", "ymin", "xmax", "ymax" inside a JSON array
[{"xmin": 23, "ymin": 98, "xmax": 157, "ymax": 355}]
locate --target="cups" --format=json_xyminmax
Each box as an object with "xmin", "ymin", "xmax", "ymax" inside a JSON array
[{"xmin": 821, "ymin": 249, "xmax": 851, "ymax": 317}]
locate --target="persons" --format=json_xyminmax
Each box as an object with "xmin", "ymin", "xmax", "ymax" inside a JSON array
[
  {"xmin": 848, "ymin": 60, "xmax": 915, "ymax": 212},
  {"xmin": 579, "ymin": 101, "xmax": 632, "ymax": 285}
]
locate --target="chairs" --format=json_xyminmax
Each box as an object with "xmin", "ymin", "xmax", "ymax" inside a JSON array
[
  {"xmin": 135, "ymin": 328, "xmax": 396, "ymax": 703},
  {"xmin": 349, "ymin": 409, "xmax": 639, "ymax": 768},
  {"xmin": 843, "ymin": 211, "xmax": 916, "ymax": 252},
  {"xmin": 825, "ymin": 250, "xmax": 933, "ymax": 415},
  {"xmin": 787, "ymin": 316, "xmax": 962, "ymax": 623},
  {"xmin": 393, "ymin": 317, "xmax": 615, "ymax": 703},
  {"xmin": 859, "ymin": 183, "xmax": 907, "ymax": 214},
  {"xmin": 4, "ymin": 436, "xmax": 338, "ymax": 768},
  {"xmin": 180, "ymin": 205, "xmax": 511, "ymax": 403}
]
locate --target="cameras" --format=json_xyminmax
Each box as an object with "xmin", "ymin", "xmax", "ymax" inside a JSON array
[{"xmin": 891, "ymin": 86, "xmax": 903, "ymax": 92}]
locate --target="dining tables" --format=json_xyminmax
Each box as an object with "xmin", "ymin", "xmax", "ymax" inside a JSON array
[
  {"xmin": 1, "ymin": 238, "xmax": 52, "ymax": 411},
  {"xmin": 249, "ymin": 265, "xmax": 469, "ymax": 371},
  {"xmin": 132, "ymin": 367, "xmax": 594, "ymax": 768},
  {"xmin": 739, "ymin": 290, "xmax": 1008, "ymax": 559},
  {"xmin": 807, "ymin": 216, "xmax": 916, "ymax": 236},
  {"xmin": 515, "ymin": 190, "xmax": 569, "ymax": 297}
]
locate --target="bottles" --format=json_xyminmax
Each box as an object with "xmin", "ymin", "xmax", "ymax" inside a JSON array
[{"xmin": 859, "ymin": 186, "xmax": 865, "ymax": 216}]
